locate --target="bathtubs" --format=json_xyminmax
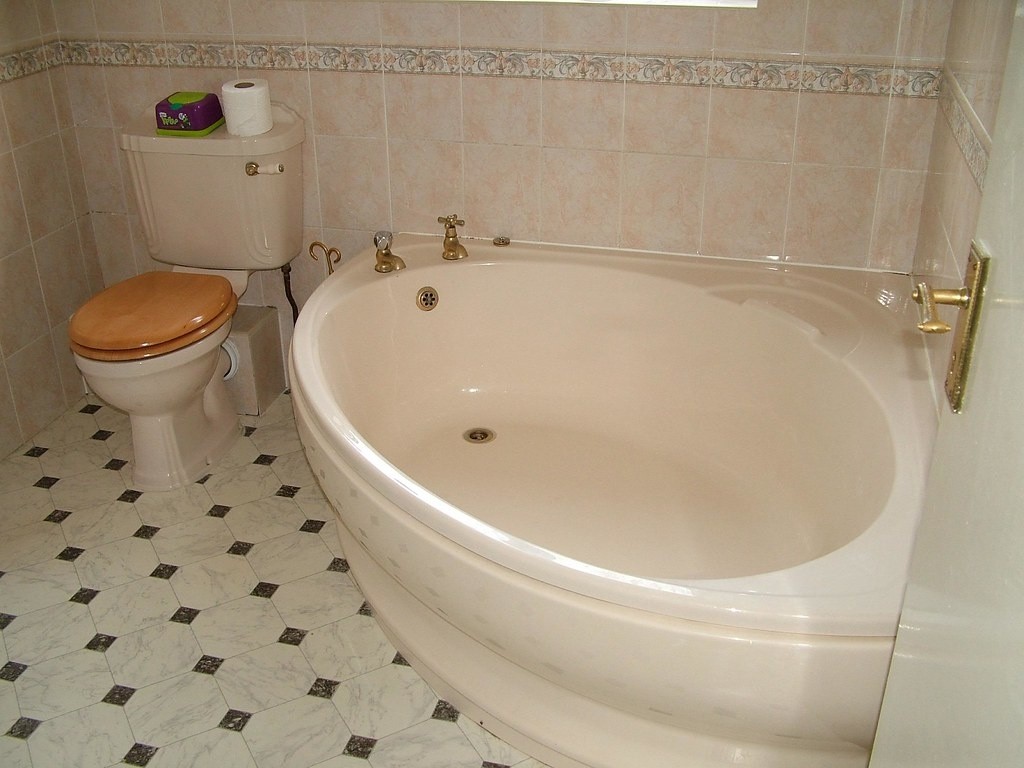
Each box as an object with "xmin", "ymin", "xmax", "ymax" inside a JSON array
[{"xmin": 288, "ymin": 234, "xmax": 940, "ymax": 766}]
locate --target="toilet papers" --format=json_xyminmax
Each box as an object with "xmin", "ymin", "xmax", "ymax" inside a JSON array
[{"xmin": 220, "ymin": 77, "xmax": 274, "ymax": 138}]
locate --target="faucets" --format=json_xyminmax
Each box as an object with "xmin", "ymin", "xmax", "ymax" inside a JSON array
[
  {"xmin": 372, "ymin": 230, "xmax": 406, "ymax": 274},
  {"xmin": 436, "ymin": 214, "xmax": 469, "ymax": 262}
]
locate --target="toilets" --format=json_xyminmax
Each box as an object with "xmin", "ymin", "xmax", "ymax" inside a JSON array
[{"xmin": 69, "ymin": 113, "xmax": 305, "ymax": 491}]
